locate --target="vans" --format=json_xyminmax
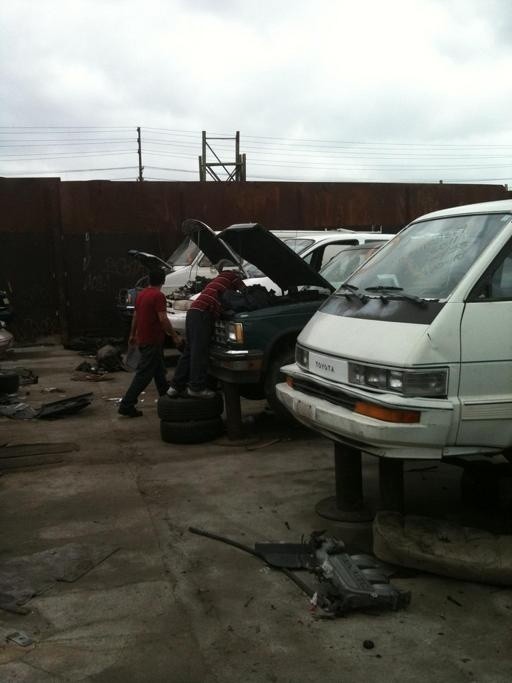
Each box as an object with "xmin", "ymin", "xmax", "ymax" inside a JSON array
[{"xmin": 277, "ymin": 199, "xmax": 512, "ymax": 459}]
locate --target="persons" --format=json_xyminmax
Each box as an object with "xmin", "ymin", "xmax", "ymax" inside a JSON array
[
  {"xmin": 118, "ymin": 269, "xmax": 181, "ymax": 418},
  {"xmin": 166, "ymin": 269, "xmax": 248, "ymax": 400}
]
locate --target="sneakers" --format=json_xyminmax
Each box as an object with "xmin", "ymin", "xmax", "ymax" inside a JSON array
[
  {"xmin": 185, "ymin": 385, "xmax": 218, "ymax": 401},
  {"xmin": 117, "ymin": 405, "xmax": 145, "ymax": 418},
  {"xmin": 165, "ymin": 384, "xmax": 187, "ymax": 398}
]
